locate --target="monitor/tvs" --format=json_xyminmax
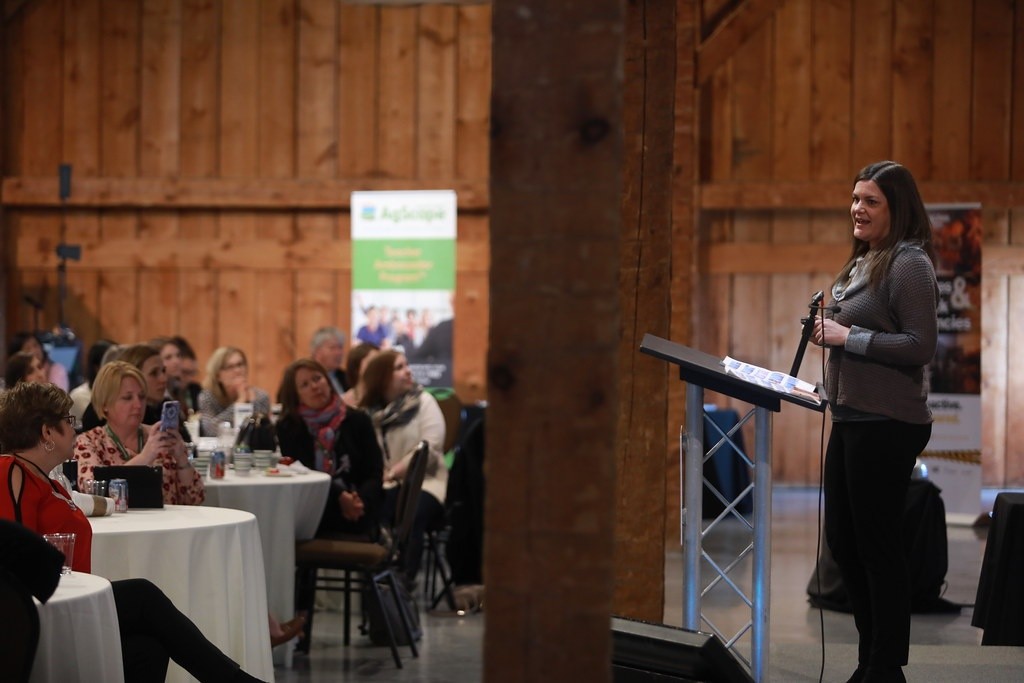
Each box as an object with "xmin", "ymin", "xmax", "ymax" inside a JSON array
[{"xmin": 610, "ymin": 615, "xmax": 752, "ymax": 683}]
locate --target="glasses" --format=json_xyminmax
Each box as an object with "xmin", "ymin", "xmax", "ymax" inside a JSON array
[{"xmin": 61, "ymin": 414, "xmax": 76, "ymax": 428}]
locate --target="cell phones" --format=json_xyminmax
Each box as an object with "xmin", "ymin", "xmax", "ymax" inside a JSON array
[{"xmin": 160, "ymin": 401, "xmax": 180, "ymax": 432}]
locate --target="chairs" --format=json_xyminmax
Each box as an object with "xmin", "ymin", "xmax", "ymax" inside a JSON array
[
  {"xmin": 293, "ymin": 440, "xmax": 430, "ymax": 670},
  {"xmin": 374, "ymin": 413, "xmax": 486, "ymax": 615},
  {"xmin": 428, "ymin": 387, "xmax": 463, "ymax": 453}
]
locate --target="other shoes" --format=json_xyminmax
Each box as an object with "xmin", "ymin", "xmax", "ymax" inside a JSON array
[
  {"xmin": 846, "ymin": 666, "xmax": 906, "ymax": 683},
  {"xmin": 271, "ymin": 616, "xmax": 311, "ymax": 646}
]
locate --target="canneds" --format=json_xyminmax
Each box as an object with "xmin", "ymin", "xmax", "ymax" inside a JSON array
[
  {"xmin": 109, "ymin": 478, "xmax": 128, "ymax": 513},
  {"xmin": 209, "ymin": 451, "xmax": 225, "ymax": 478}
]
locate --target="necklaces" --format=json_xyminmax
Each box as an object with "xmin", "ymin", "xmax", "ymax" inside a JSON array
[{"xmin": 10, "ymin": 452, "xmax": 77, "ymax": 511}]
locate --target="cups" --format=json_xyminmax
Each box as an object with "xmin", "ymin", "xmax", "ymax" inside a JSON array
[
  {"xmin": 234, "ymin": 453, "xmax": 252, "ymax": 476},
  {"xmin": 193, "ymin": 458, "xmax": 210, "ymax": 481},
  {"xmin": 44, "ymin": 532, "xmax": 76, "ymax": 576},
  {"xmin": 84, "ymin": 480, "xmax": 106, "ymax": 496},
  {"xmin": 73, "ymin": 491, "xmax": 115, "ymax": 517},
  {"xmin": 254, "ymin": 450, "xmax": 271, "ymax": 473},
  {"xmin": 182, "ymin": 419, "xmax": 240, "ymax": 468}
]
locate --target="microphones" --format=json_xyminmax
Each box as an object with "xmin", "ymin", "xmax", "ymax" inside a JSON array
[{"xmin": 808, "ymin": 303, "xmax": 842, "ymax": 313}]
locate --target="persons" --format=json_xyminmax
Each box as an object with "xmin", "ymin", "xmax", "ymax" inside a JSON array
[
  {"xmin": 357, "ymin": 300, "xmax": 436, "ymax": 360},
  {"xmin": 275, "ymin": 325, "xmax": 486, "ymax": 643},
  {"xmin": 801, "ymin": 158, "xmax": 940, "ymax": 683},
  {"xmin": 0, "ymin": 329, "xmax": 274, "ymax": 683}
]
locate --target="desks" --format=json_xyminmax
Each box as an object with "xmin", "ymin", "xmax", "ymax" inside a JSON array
[
  {"xmin": 68, "ymin": 504, "xmax": 257, "ymax": 683},
  {"xmin": 30, "ymin": 571, "xmax": 113, "ymax": 683},
  {"xmin": 169, "ymin": 470, "xmax": 332, "ymax": 624}
]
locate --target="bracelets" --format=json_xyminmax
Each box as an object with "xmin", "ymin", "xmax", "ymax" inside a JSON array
[{"xmin": 174, "ymin": 461, "xmax": 190, "ymax": 469}]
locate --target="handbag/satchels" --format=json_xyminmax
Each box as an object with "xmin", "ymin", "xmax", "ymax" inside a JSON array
[
  {"xmin": 368, "ymin": 577, "xmax": 424, "ymax": 643},
  {"xmin": 93, "ymin": 464, "xmax": 163, "ymax": 508}
]
